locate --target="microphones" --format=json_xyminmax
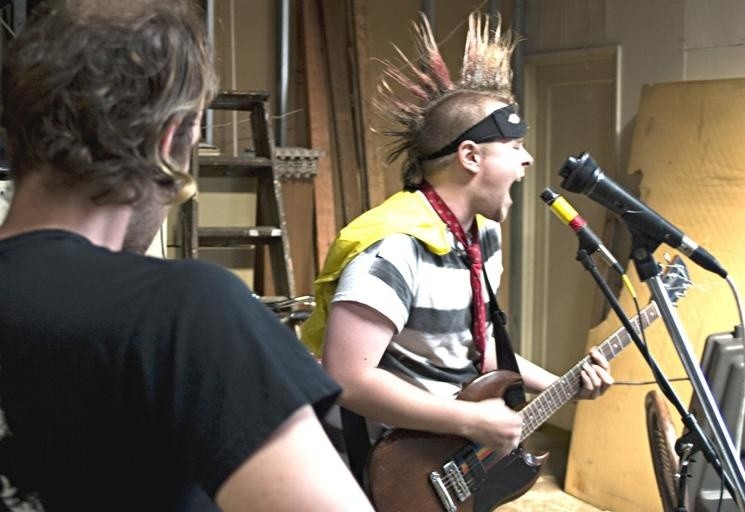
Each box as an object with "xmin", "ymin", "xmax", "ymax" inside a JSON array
[
  {"xmin": 557, "ymin": 149, "xmax": 727, "ymax": 279},
  {"xmin": 540, "ymin": 186, "xmax": 624, "ymax": 276}
]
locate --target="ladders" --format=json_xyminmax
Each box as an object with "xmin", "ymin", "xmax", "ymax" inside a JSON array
[{"xmin": 172, "ymin": 91, "xmax": 300, "ymax": 344}]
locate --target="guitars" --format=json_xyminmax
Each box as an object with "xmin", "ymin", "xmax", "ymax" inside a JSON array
[{"xmin": 369, "ymin": 253, "xmax": 694, "ymax": 512}]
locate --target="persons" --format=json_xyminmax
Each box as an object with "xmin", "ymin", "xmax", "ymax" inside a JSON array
[
  {"xmin": 297, "ymin": 8, "xmax": 619, "ymax": 453},
  {"xmin": 0, "ymin": 1, "xmax": 378, "ymax": 512}
]
